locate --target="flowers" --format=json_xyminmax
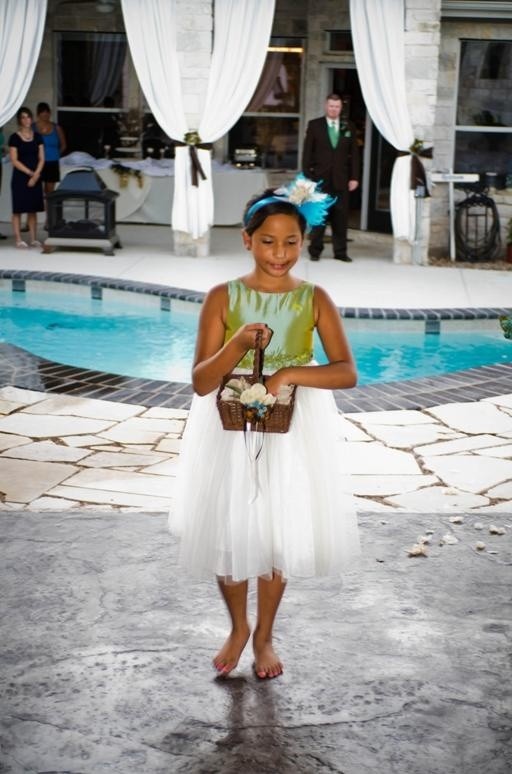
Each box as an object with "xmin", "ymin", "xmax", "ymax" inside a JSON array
[{"xmin": 225, "ymin": 374, "xmax": 275, "ymax": 421}]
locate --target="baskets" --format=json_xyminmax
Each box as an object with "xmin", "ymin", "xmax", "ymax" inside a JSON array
[{"xmin": 217, "ymin": 328, "xmax": 294, "ymax": 432}]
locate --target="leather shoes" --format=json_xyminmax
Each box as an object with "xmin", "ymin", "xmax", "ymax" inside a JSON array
[{"xmin": 310, "ymin": 254, "xmax": 352, "ymax": 262}]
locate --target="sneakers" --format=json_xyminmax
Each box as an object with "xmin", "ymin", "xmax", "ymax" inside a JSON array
[{"xmin": 17, "ymin": 240, "xmax": 41, "ymax": 248}]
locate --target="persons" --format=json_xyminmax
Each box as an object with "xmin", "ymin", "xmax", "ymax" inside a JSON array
[
  {"xmin": 9, "ymin": 107, "xmax": 45, "ymax": 249},
  {"xmin": 0, "ymin": 125, "xmax": 7, "ymax": 240},
  {"xmin": 167, "ymin": 171, "xmax": 361, "ymax": 679},
  {"xmin": 302, "ymin": 92, "xmax": 361, "ymax": 262},
  {"xmin": 21, "ymin": 101, "xmax": 67, "ymax": 233}
]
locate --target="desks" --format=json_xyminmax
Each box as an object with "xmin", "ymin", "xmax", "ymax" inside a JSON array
[{"xmin": 0, "ymin": 158, "xmax": 267, "ymax": 227}]
